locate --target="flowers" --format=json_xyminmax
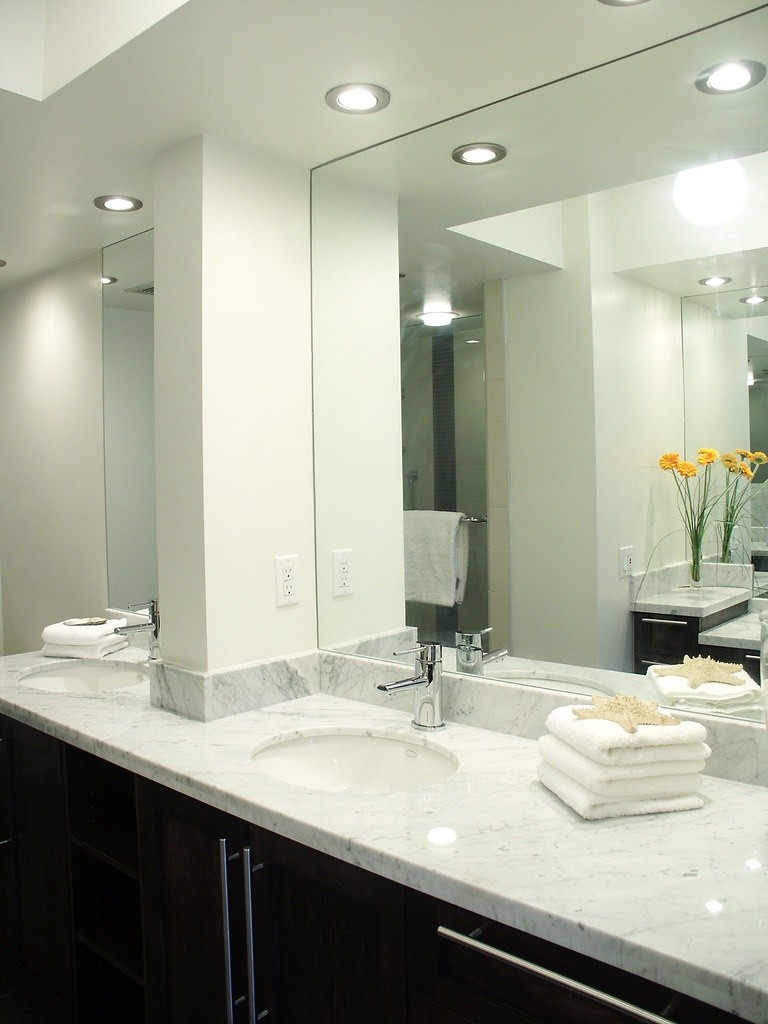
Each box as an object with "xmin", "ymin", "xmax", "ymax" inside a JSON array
[
  {"xmin": 660, "ymin": 444, "xmax": 719, "ymax": 583},
  {"xmin": 719, "ymin": 449, "xmax": 768, "ymax": 564}
]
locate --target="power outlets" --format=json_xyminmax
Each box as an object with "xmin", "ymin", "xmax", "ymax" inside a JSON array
[
  {"xmin": 272, "ymin": 555, "xmax": 301, "ymax": 608},
  {"xmin": 330, "ymin": 548, "xmax": 356, "ymax": 598},
  {"xmin": 618, "ymin": 546, "xmax": 633, "ymax": 579}
]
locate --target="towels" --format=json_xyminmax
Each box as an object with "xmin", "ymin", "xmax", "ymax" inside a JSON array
[
  {"xmin": 537, "ymin": 760, "xmax": 706, "ymax": 820},
  {"xmin": 403, "ymin": 510, "xmax": 469, "ymax": 608},
  {"xmin": 42, "ymin": 634, "xmax": 128, "ymax": 659},
  {"xmin": 41, "ymin": 618, "xmax": 128, "ymax": 645},
  {"xmin": 707, "ymin": 705, "xmax": 764, "ymax": 722},
  {"xmin": 645, "ymin": 665, "xmax": 763, "ymax": 707},
  {"xmin": 536, "ymin": 734, "xmax": 712, "ymax": 796},
  {"xmin": 545, "ymin": 704, "xmax": 712, "ymax": 766}
]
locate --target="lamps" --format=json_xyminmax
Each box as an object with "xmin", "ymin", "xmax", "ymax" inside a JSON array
[{"xmin": 415, "ymin": 311, "xmax": 460, "ymax": 327}]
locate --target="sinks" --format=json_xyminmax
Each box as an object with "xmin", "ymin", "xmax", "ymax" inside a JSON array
[
  {"xmin": 484, "ymin": 671, "xmax": 619, "ymax": 697},
  {"xmin": 248, "ymin": 727, "xmax": 460, "ymax": 794}
]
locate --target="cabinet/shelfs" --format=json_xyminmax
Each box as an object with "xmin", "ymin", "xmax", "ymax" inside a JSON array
[
  {"xmin": 751, "ymin": 556, "xmax": 768, "ymax": 572},
  {"xmin": 634, "ymin": 601, "xmax": 761, "ymax": 686},
  {"xmin": 0, "ymin": 713, "xmax": 753, "ymax": 1024}
]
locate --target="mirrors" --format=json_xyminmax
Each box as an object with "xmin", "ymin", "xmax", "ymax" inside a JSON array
[
  {"xmin": 680, "ymin": 286, "xmax": 768, "ymax": 600},
  {"xmin": 309, "ymin": 4, "xmax": 768, "ymax": 725},
  {"xmin": 101, "ymin": 228, "xmax": 159, "ymax": 652}
]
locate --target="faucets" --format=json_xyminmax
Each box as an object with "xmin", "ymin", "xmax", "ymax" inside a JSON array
[
  {"xmin": 114, "ymin": 599, "xmax": 160, "ymax": 660},
  {"xmin": 376, "ymin": 640, "xmax": 446, "ymax": 732},
  {"xmin": 455, "ymin": 625, "xmax": 509, "ymax": 676}
]
